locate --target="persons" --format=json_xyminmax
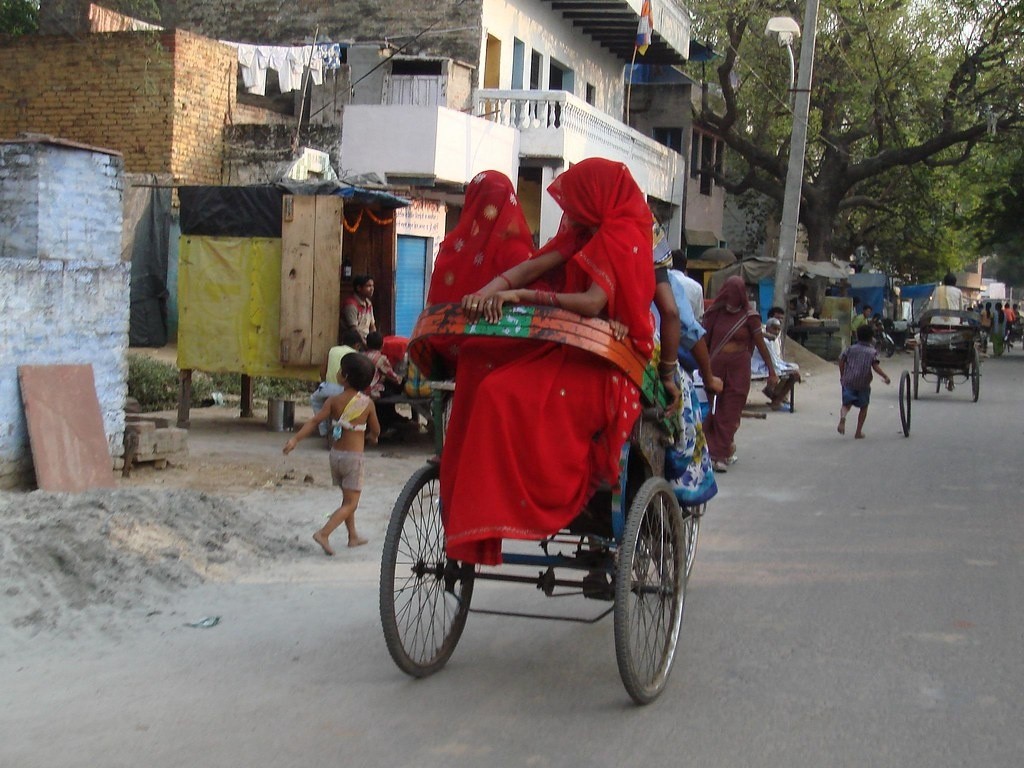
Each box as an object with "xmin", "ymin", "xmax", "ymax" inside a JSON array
[
  {"xmin": 786, "ymin": 293, "xmax": 810, "ymax": 347},
  {"xmin": 701, "ymin": 275, "xmax": 780, "ymax": 473},
  {"xmin": 930, "ymin": 273, "xmax": 964, "ymax": 330},
  {"xmin": 851, "ymin": 295, "xmax": 882, "ymax": 356},
  {"xmin": 966, "ymin": 300, "xmax": 1024, "ymax": 358},
  {"xmin": 837, "ymin": 325, "xmax": 890, "ymax": 439},
  {"xmin": 426, "ymin": 170, "xmax": 629, "ymax": 341},
  {"xmin": 666, "ymin": 248, "xmax": 706, "ymax": 323},
  {"xmin": 309, "ymin": 333, "xmax": 364, "ymax": 450},
  {"xmin": 282, "ymin": 353, "xmax": 380, "ymax": 555},
  {"xmin": 439, "ymin": 158, "xmax": 657, "ymax": 566},
  {"xmin": 339, "ymin": 273, "xmax": 378, "ymax": 352},
  {"xmin": 362, "ymin": 332, "xmax": 408, "ymax": 401},
  {"xmin": 655, "ymin": 266, "xmax": 682, "ymax": 418},
  {"xmin": 750, "ymin": 307, "xmax": 802, "ymax": 411}
]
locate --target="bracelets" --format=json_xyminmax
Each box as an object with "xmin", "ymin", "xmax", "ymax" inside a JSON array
[
  {"xmin": 658, "ymin": 369, "xmax": 677, "ymax": 377},
  {"xmin": 498, "ymin": 274, "xmax": 512, "ymax": 290},
  {"xmin": 535, "ymin": 289, "xmax": 560, "ymax": 308},
  {"xmin": 472, "ymin": 292, "xmax": 482, "ymax": 296},
  {"xmin": 659, "ymin": 356, "xmax": 678, "ymax": 366}
]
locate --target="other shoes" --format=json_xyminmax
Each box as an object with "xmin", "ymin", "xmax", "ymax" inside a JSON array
[
  {"xmin": 726, "ymin": 455, "xmax": 738, "ymax": 465},
  {"xmin": 714, "ymin": 461, "xmax": 728, "ymax": 472},
  {"xmin": 948, "ymin": 381, "xmax": 954, "ymax": 391}
]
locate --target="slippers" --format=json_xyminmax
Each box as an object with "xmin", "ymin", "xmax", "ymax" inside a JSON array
[
  {"xmin": 772, "ymin": 402, "xmax": 791, "ymax": 411},
  {"xmin": 762, "ymin": 387, "xmax": 775, "ymax": 401}
]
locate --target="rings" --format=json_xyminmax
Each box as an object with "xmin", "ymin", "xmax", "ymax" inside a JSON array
[
  {"xmin": 487, "ymin": 300, "xmax": 492, "ymax": 304},
  {"xmin": 471, "ymin": 303, "xmax": 478, "ymax": 308}
]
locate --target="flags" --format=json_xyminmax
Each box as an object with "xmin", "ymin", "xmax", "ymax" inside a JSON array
[{"xmin": 634, "ymin": 0, "xmax": 653, "ymax": 55}]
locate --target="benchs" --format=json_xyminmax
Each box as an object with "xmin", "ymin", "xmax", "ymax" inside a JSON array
[
  {"xmin": 751, "ymin": 371, "xmax": 795, "ymax": 413},
  {"xmin": 919, "ymin": 324, "xmax": 977, "ymax": 369},
  {"xmin": 406, "ymin": 301, "xmax": 719, "ymax": 538}
]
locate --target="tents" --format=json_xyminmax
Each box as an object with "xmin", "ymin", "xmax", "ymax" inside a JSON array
[{"xmin": 899, "ymin": 282, "xmax": 983, "ymax": 324}]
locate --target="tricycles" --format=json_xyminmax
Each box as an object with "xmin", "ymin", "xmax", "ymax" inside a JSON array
[
  {"xmin": 376, "ymin": 298, "xmax": 726, "ymax": 664},
  {"xmin": 905, "ymin": 307, "xmax": 986, "ymax": 404}
]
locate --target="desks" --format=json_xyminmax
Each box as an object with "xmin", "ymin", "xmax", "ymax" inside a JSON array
[{"xmin": 790, "ymin": 325, "xmax": 840, "ymax": 361}]
